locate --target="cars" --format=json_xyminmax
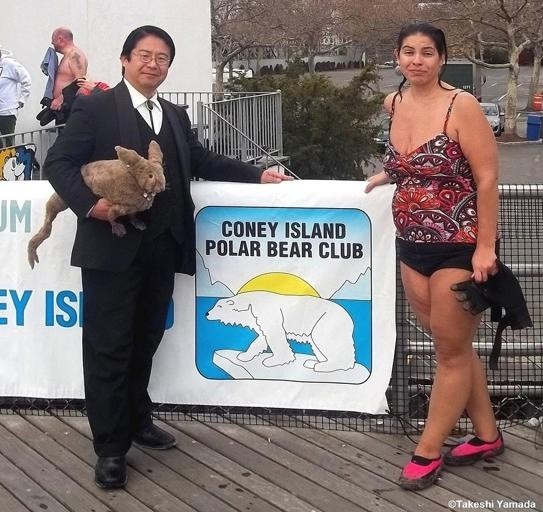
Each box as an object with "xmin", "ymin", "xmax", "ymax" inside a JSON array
[
  {"xmin": 478, "ymin": 102, "xmax": 505, "ymax": 136},
  {"xmin": 385, "ymin": 61, "xmax": 394, "ymax": 66},
  {"xmin": 373, "ymin": 118, "xmax": 390, "ymax": 150}
]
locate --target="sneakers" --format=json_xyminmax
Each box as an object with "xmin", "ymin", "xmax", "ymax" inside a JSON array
[
  {"xmin": 397, "ymin": 453, "xmax": 446, "ymax": 492},
  {"xmin": 442, "ymin": 429, "xmax": 506, "ymax": 466}
]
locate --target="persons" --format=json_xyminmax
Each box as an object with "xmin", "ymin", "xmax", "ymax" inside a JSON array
[
  {"xmin": 0, "ymin": 45, "xmax": 32, "ymax": 149},
  {"xmin": 74, "ymin": 75, "xmax": 112, "ymax": 97},
  {"xmin": 361, "ymin": 23, "xmax": 505, "ymax": 490},
  {"xmin": 49, "ymin": 27, "xmax": 88, "ymax": 124},
  {"xmin": 41, "ymin": 23, "xmax": 297, "ymax": 491}
]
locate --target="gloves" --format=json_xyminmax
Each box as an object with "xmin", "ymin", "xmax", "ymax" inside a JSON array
[{"xmin": 450, "ymin": 258, "xmax": 534, "ymax": 330}]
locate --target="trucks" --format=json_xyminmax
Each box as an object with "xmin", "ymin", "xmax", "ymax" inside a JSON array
[{"xmin": 440, "ymin": 59, "xmax": 486, "ymax": 102}]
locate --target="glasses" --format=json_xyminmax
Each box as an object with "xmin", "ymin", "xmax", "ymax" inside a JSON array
[{"xmin": 131, "ymin": 51, "xmax": 171, "ymax": 66}]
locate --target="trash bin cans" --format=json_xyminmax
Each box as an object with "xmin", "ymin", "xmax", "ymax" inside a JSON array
[{"xmin": 527, "ymin": 113, "xmax": 543, "ymax": 141}]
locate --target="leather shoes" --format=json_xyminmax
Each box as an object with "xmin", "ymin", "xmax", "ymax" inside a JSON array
[
  {"xmin": 93, "ymin": 450, "xmax": 130, "ymax": 487},
  {"xmin": 130, "ymin": 423, "xmax": 179, "ymax": 452}
]
